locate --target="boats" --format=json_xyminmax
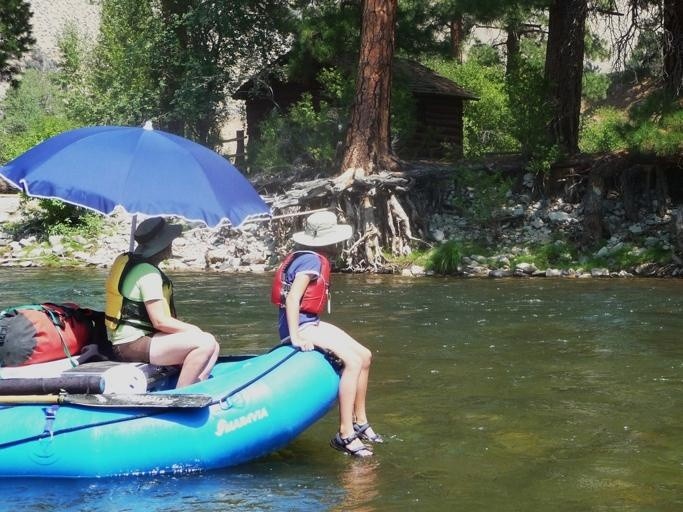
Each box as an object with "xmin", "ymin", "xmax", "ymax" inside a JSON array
[{"xmin": 0, "ymin": 339, "xmax": 342, "ymax": 480}]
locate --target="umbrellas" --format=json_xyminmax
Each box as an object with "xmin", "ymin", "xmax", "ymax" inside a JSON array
[{"xmin": 0, "ymin": 121, "xmax": 272, "ymax": 253}]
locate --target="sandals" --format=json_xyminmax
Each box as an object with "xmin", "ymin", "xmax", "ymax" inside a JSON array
[{"xmin": 329, "ymin": 422, "xmax": 384, "ymax": 458}]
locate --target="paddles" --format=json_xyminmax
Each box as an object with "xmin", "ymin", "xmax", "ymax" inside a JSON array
[{"xmin": 0, "ymin": 393, "xmax": 211, "ymax": 409}]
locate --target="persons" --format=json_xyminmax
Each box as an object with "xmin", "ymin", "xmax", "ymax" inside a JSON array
[
  {"xmin": 104, "ymin": 218, "xmax": 220, "ymax": 389},
  {"xmin": 277, "ymin": 211, "xmax": 384, "ymax": 460}
]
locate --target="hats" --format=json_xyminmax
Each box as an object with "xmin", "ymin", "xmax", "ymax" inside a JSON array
[
  {"xmin": 293, "ymin": 211, "xmax": 353, "ymax": 247},
  {"xmin": 133, "ymin": 216, "xmax": 183, "ymax": 259}
]
